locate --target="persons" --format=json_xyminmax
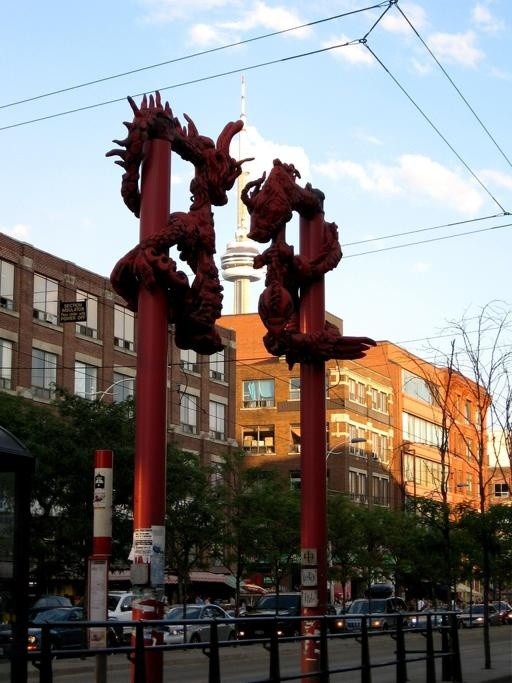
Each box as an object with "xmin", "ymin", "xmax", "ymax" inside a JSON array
[
  {"xmin": 194, "ymin": 592, "xmax": 204, "ymax": 603},
  {"xmin": 202, "ymin": 595, "xmax": 212, "ymax": 605}
]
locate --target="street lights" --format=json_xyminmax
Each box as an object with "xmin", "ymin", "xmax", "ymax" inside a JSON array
[{"xmin": 327, "ymin": 435, "xmax": 370, "ymax": 459}]
